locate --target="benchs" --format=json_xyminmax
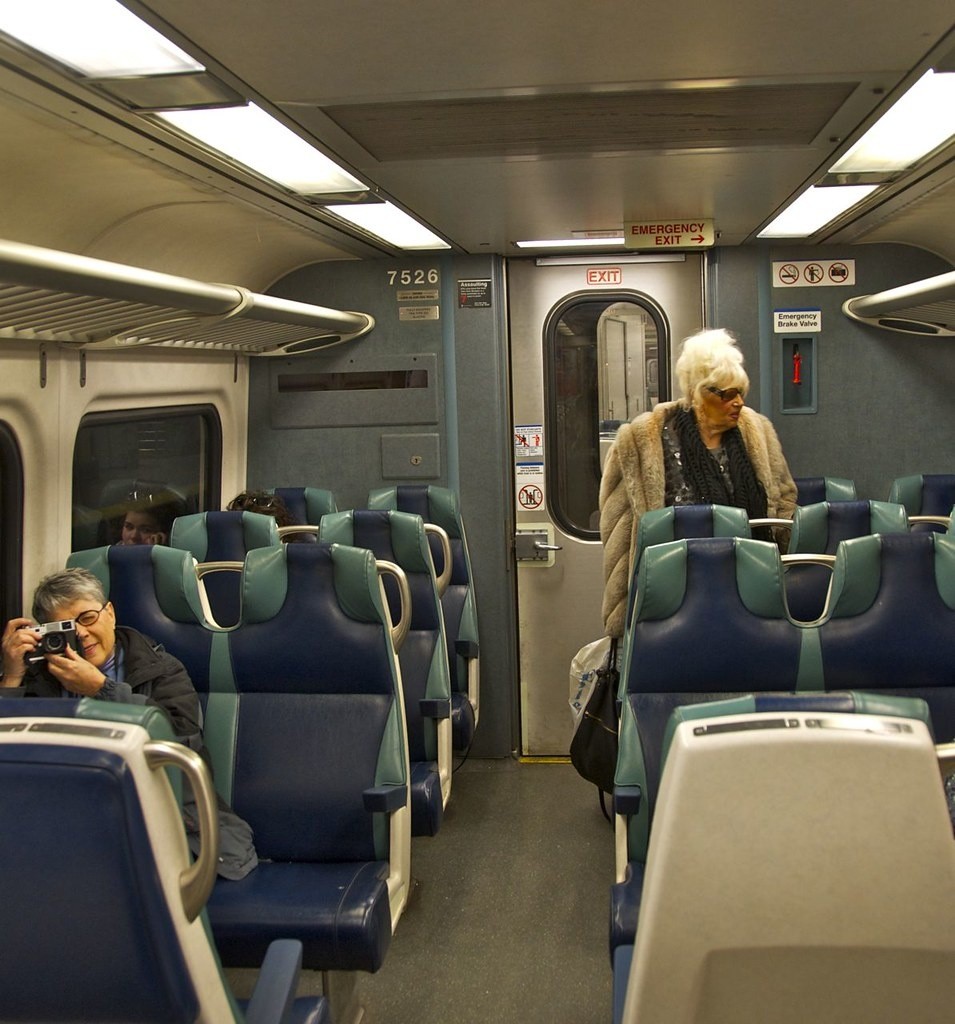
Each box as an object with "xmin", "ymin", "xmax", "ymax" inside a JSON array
[
  {"xmin": 53, "ymin": 541, "xmax": 417, "ymax": 1024},
  {"xmin": 259, "ymin": 485, "xmax": 481, "ymax": 751},
  {"xmin": 170, "ymin": 510, "xmax": 453, "ymax": 913},
  {"xmin": 609, "ymin": 499, "xmax": 954, "ymax": 1024},
  {"xmin": 887, "ymin": 474, "xmax": 955, "ymax": 538}
]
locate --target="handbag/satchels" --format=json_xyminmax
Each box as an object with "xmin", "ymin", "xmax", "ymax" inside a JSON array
[{"xmin": 568, "ymin": 635, "xmax": 620, "ymax": 823}]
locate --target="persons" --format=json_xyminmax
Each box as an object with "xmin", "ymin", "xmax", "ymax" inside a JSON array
[
  {"xmin": 1, "ymin": 567, "xmax": 262, "ymax": 884},
  {"xmin": 117, "ymin": 486, "xmax": 182, "ymax": 548},
  {"xmin": 219, "ymin": 489, "xmax": 317, "ymax": 543},
  {"xmin": 595, "ymin": 327, "xmax": 799, "ymax": 639}
]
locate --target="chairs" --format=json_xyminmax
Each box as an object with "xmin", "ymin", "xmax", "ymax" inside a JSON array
[{"xmin": 0, "ymin": 698, "xmax": 328, "ymax": 1024}]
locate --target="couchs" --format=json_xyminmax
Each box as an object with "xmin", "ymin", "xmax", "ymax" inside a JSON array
[{"xmin": 797, "ymin": 476, "xmax": 857, "ymax": 506}]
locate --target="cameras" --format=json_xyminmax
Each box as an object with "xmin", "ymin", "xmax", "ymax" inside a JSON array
[{"xmin": 22, "ymin": 619, "xmax": 84, "ymax": 667}]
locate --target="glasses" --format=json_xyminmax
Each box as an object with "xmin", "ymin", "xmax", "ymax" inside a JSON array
[
  {"xmin": 75, "ymin": 605, "xmax": 105, "ymax": 626},
  {"xmin": 703, "ymin": 384, "xmax": 741, "ymax": 401},
  {"xmin": 234, "ymin": 494, "xmax": 277, "ymax": 508}
]
